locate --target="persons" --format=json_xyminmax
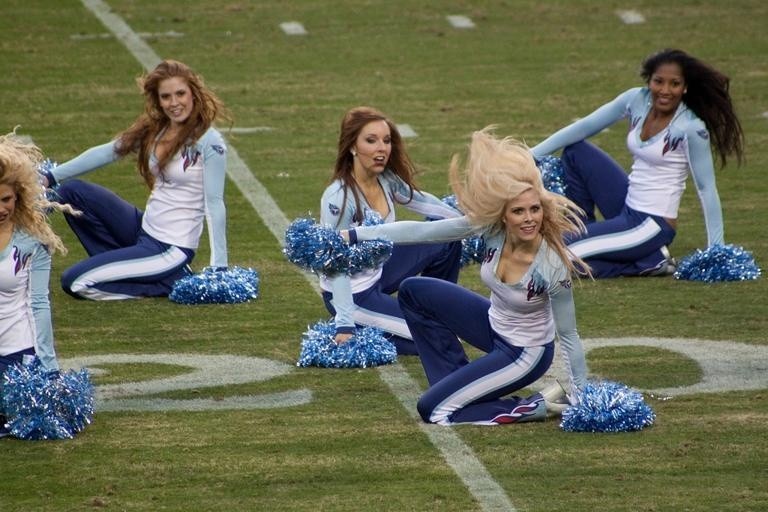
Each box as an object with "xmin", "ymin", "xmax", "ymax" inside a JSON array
[
  {"xmin": 527, "ymin": 47, "xmax": 745, "ymax": 279},
  {"xmin": 317, "ymin": 106, "xmax": 467, "ymax": 353},
  {"xmin": 36, "ymin": 62, "xmax": 230, "ymax": 304},
  {"xmin": 332, "ymin": 124, "xmax": 594, "ymax": 424},
  {"xmin": 1, "ymin": 128, "xmax": 82, "ymax": 436}
]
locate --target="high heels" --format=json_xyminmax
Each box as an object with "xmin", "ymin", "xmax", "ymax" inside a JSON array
[{"xmin": 540, "ymin": 382, "xmax": 570, "ymax": 415}]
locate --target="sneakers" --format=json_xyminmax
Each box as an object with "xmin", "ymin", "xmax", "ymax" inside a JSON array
[{"xmin": 660, "ymin": 245, "xmax": 678, "ymax": 273}]
